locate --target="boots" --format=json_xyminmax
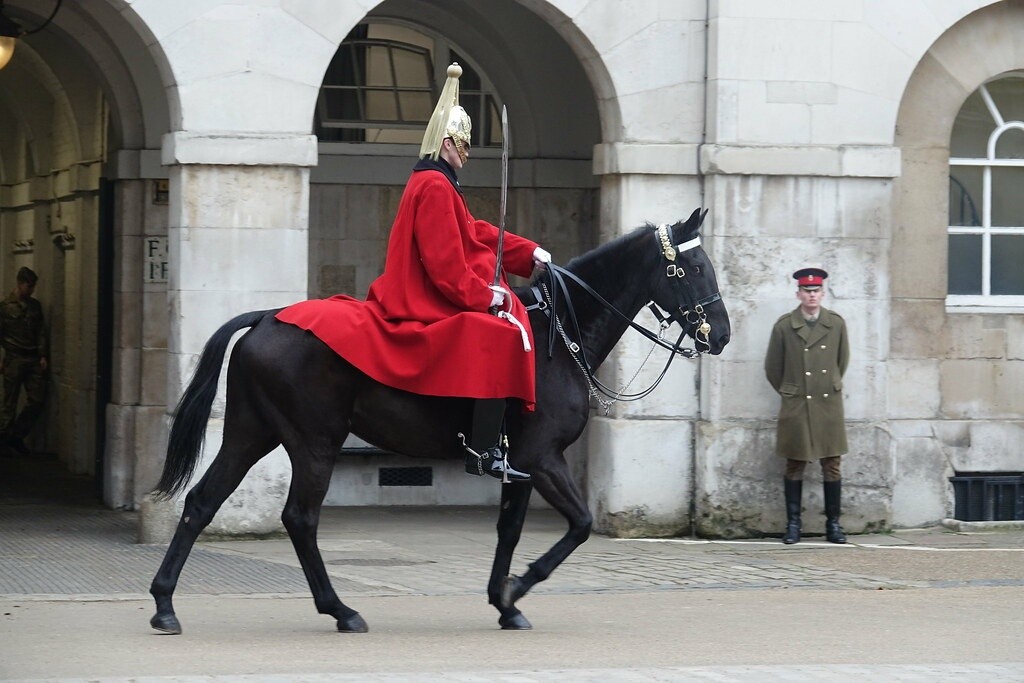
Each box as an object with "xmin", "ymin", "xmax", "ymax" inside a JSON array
[
  {"xmin": 823, "ymin": 481, "xmax": 844, "ymax": 544},
  {"xmin": 783, "ymin": 477, "xmax": 803, "ymax": 545}
]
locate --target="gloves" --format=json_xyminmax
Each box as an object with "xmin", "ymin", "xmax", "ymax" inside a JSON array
[
  {"xmin": 489, "ymin": 285, "xmax": 505, "ymax": 306},
  {"xmin": 534, "ymin": 245, "xmax": 551, "ymax": 271}
]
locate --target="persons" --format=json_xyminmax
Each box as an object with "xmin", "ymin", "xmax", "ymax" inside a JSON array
[
  {"xmin": 274, "ymin": 105, "xmax": 552, "ymax": 480},
  {"xmin": 0, "ymin": 267, "xmax": 49, "ymax": 457},
  {"xmin": 766, "ymin": 269, "xmax": 848, "ymax": 544}
]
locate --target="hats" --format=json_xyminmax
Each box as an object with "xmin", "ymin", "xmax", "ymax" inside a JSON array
[{"xmin": 792, "ymin": 268, "xmax": 828, "ymax": 290}]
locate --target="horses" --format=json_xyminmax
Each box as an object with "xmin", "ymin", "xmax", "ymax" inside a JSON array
[{"xmin": 143, "ymin": 205, "xmax": 732, "ymax": 635}]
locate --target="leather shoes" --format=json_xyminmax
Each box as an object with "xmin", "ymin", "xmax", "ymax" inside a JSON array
[
  {"xmin": 465, "ymin": 432, "xmax": 531, "ymax": 481},
  {"xmin": 1, "ymin": 438, "xmax": 32, "ymax": 457}
]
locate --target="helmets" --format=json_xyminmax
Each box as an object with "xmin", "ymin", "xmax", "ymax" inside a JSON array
[{"xmin": 419, "ymin": 61, "xmax": 472, "ymax": 165}]
